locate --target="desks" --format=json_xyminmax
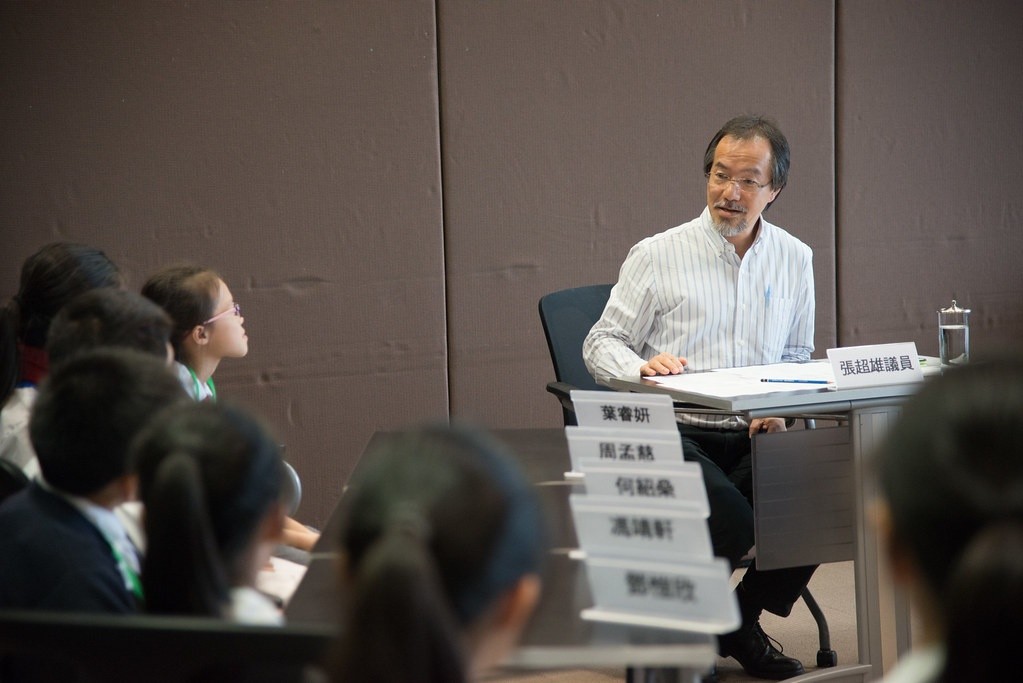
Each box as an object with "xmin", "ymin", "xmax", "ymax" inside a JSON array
[{"xmin": 593, "ymin": 355, "xmax": 952, "ymax": 683}]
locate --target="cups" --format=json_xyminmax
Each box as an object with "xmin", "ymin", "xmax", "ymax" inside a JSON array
[{"xmin": 938, "ymin": 310, "xmax": 970, "ymax": 367}]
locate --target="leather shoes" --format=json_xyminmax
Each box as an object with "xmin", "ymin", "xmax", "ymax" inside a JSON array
[{"xmin": 716, "ymin": 587, "xmax": 804, "ymax": 679}]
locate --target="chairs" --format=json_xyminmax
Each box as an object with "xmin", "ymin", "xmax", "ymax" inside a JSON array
[
  {"xmin": 0, "ymin": 611, "xmax": 340, "ymax": 683},
  {"xmin": 538, "ymin": 284, "xmax": 836, "ymax": 683}
]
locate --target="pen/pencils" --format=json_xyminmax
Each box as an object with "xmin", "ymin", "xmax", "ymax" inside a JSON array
[{"xmin": 760, "ymin": 377, "xmax": 835, "ymax": 384}]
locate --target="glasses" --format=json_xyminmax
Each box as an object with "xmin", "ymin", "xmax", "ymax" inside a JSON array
[
  {"xmin": 705, "ymin": 170, "xmax": 773, "ymax": 194},
  {"xmin": 181, "ymin": 303, "xmax": 240, "ymax": 338}
]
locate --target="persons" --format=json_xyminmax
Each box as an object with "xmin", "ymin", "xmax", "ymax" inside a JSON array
[
  {"xmin": 582, "ymin": 112, "xmax": 816, "ymax": 681},
  {"xmin": 854, "ymin": 346, "xmax": 1023, "ymax": 683},
  {"xmin": 334, "ymin": 424, "xmax": 557, "ymax": 683},
  {"xmin": 0, "ymin": 241, "xmax": 321, "ymax": 629}
]
